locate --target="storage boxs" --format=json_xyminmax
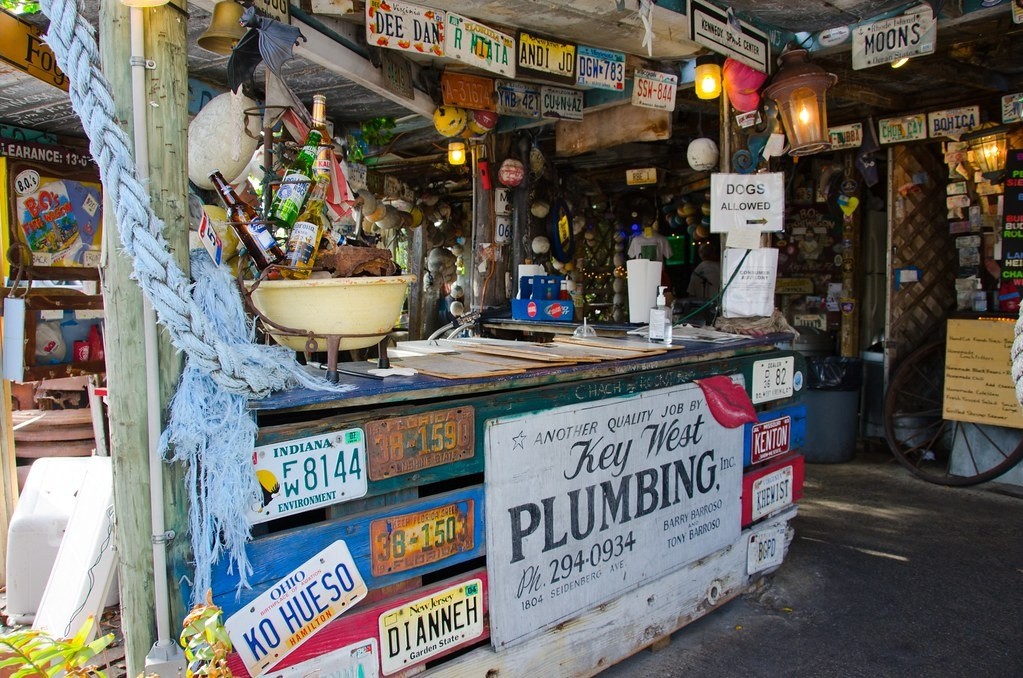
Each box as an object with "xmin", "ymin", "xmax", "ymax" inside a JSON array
[{"xmin": 512, "ymin": 298, "xmax": 575, "ymax": 321}]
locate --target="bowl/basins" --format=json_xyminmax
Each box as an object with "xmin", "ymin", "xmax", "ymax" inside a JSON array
[{"xmin": 245, "ymin": 273, "xmax": 418, "ymax": 351}]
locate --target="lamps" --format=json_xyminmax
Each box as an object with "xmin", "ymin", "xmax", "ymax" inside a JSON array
[
  {"xmin": 755, "ymin": 46, "xmax": 838, "ymax": 159},
  {"xmin": 695, "ymin": 54, "xmax": 722, "ymax": 100},
  {"xmin": 962, "ymin": 115, "xmax": 1010, "ymax": 184},
  {"xmin": 447, "ymin": 136, "xmax": 465, "ymax": 166}
]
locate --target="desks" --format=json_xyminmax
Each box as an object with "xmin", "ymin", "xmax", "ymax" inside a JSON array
[
  {"xmin": 173, "ymin": 334, "xmax": 802, "ymax": 678},
  {"xmin": 858, "ymin": 360, "xmax": 886, "ymax": 447}
]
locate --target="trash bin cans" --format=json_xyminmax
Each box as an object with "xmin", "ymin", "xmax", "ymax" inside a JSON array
[{"xmin": 801, "ymin": 356, "xmax": 863, "ymax": 463}]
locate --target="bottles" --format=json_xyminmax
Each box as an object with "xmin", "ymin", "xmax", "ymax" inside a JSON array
[
  {"xmin": 304, "ymin": 94, "xmax": 330, "ymax": 194},
  {"xmin": 546, "ymin": 288, "xmax": 552, "ymax": 300},
  {"xmin": 266, "ymin": 130, "xmax": 323, "ymax": 228},
  {"xmin": 207, "ymin": 169, "xmax": 283, "ymax": 270},
  {"xmin": 279, "ymin": 174, "xmax": 330, "ymax": 279}
]
